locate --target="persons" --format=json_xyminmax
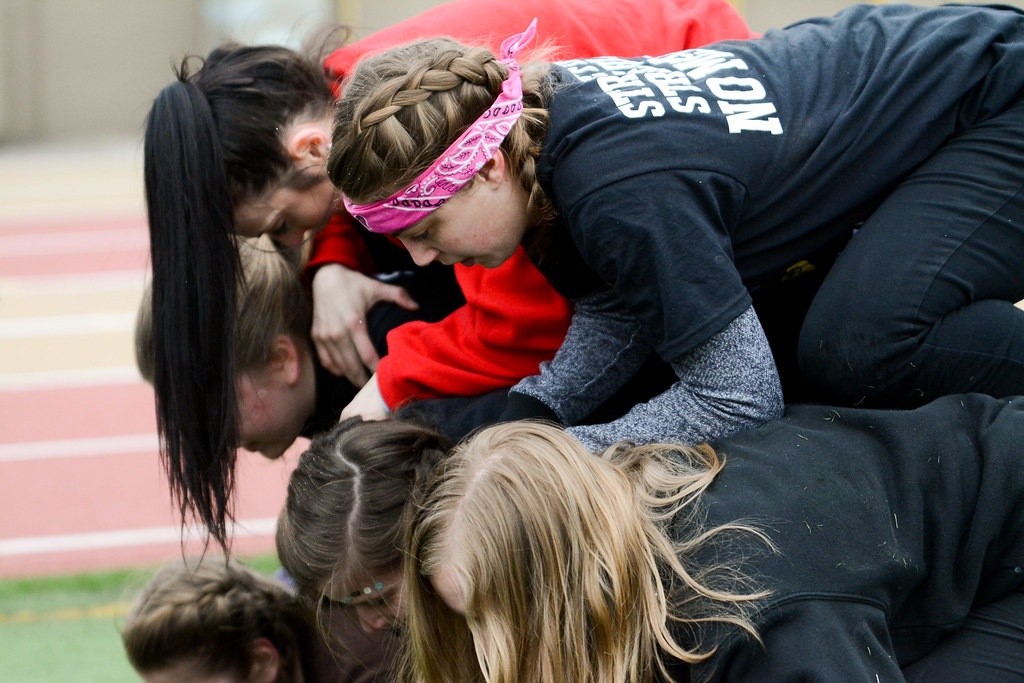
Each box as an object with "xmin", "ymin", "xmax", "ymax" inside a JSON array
[{"xmin": 116, "ymin": 0, "xmax": 1024, "ymax": 683}]
